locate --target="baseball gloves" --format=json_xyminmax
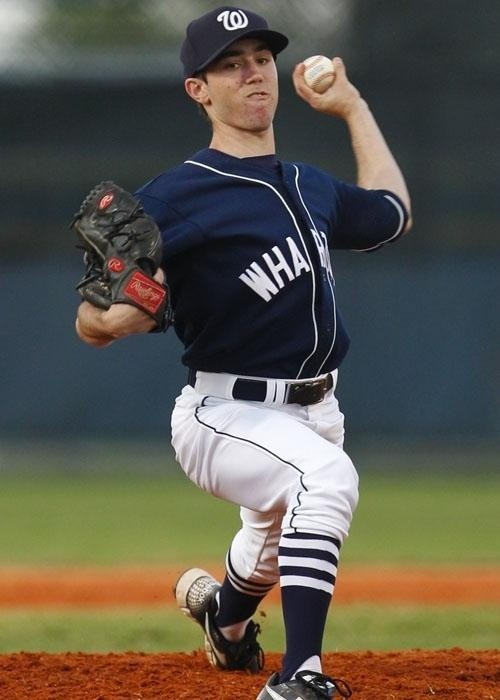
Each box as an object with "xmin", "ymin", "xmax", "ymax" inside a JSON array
[{"xmin": 65, "ymin": 178, "xmax": 173, "ymax": 337}]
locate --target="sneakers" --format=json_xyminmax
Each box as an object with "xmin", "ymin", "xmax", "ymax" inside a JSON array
[
  {"xmin": 173, "ymin": 567, "xmax": 264, "ymax": 672},
  {"xmin": 255, "ymin": 671, "xmax": 353, "ymax": 700}
]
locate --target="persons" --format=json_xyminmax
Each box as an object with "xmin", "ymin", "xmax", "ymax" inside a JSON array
[{"xmin": 72, "ymin": 5, "xmax": 417, "ymax": 700}]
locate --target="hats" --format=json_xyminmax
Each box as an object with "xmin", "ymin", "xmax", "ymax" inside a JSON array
[{"xmin": 180, "ymin": 6, "xmax": 289, "ymax": 77}]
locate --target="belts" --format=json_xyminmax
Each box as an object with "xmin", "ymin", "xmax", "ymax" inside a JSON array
[{"xmin": 186, "ymin": 368, "xmax": 334, "ymax": 406}]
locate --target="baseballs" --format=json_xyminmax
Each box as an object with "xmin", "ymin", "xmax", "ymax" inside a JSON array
[{"xmin": 301, "ymin": 54, "xmax": 338, "ymax": 94}]
